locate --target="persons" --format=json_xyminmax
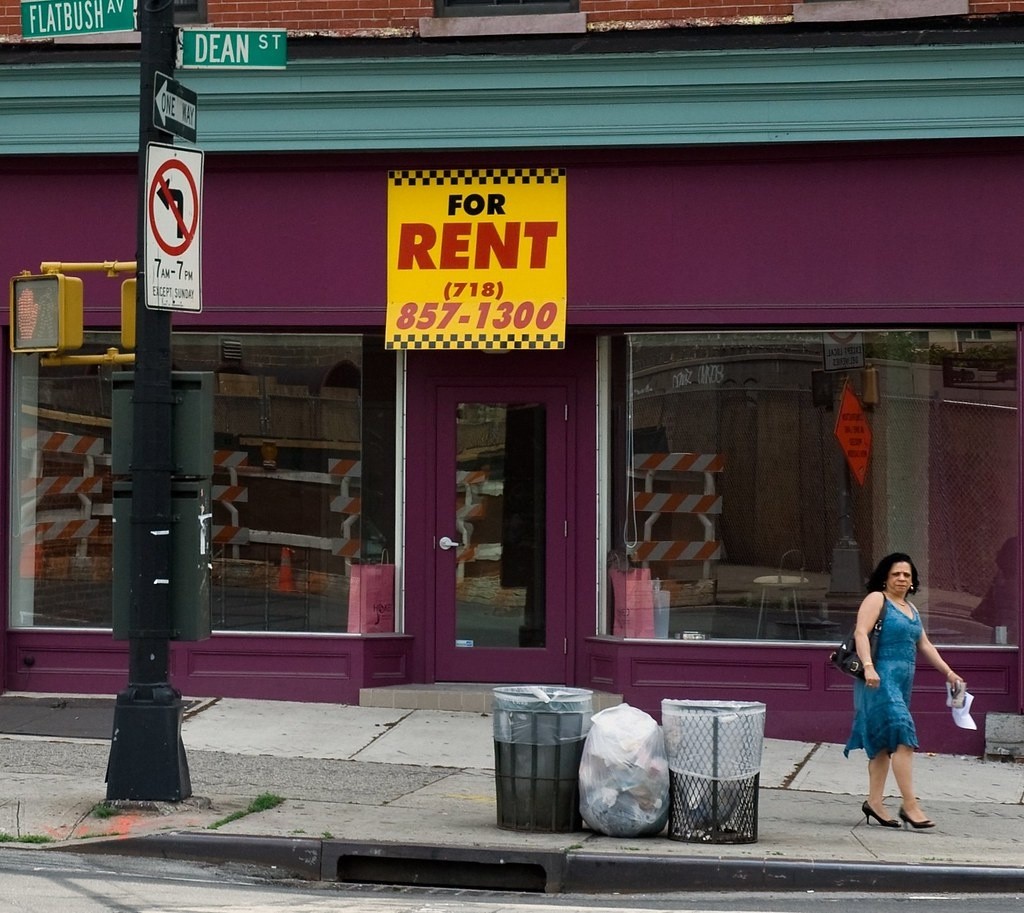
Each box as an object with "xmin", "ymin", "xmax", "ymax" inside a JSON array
[{"xmin": 853, "ymin": 552, "xmax": 965, "ymax": 829}]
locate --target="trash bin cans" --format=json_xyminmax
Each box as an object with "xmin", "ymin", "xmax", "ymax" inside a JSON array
[
  {"xmin": 660, "ymin": 694, "xmax": 765, "ymax": 845},
  {"xmin": 491, "ymin": 683, "xmax": 596, "ymax": 837}
]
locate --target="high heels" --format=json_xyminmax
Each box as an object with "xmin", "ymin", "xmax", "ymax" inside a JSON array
[
  {"xmin": 862, "ymin": 801, "xmax": 902, "ymax": 828},
  {"xmin": 899, "ymin": 807, "xmax": 935, "ymax": 830}
]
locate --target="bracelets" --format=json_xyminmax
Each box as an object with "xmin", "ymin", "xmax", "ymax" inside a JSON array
[
  {"xmin": 946, "ymin": 670, "xmax": 954, "ymax": 676},
  {"xmin": 863, "ymin": 662, "xmax": 874, "ymax": 668}
]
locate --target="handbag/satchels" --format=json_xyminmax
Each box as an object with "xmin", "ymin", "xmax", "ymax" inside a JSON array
[
  {"xmin": 609, "ymin": 552, "xmax": 656, "ymax": 641},
  {"xmin": 829, "ymin": 592, "xmax": 887, "ymax": 680},
  {"xmin": 347, "ymin": 547, "xmax": 394, "ymax": 635}
]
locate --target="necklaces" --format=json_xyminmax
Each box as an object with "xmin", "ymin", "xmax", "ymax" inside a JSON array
[{"xmin": 886, "ymin": 590, "xmax": 906, "ymax": 606}]
